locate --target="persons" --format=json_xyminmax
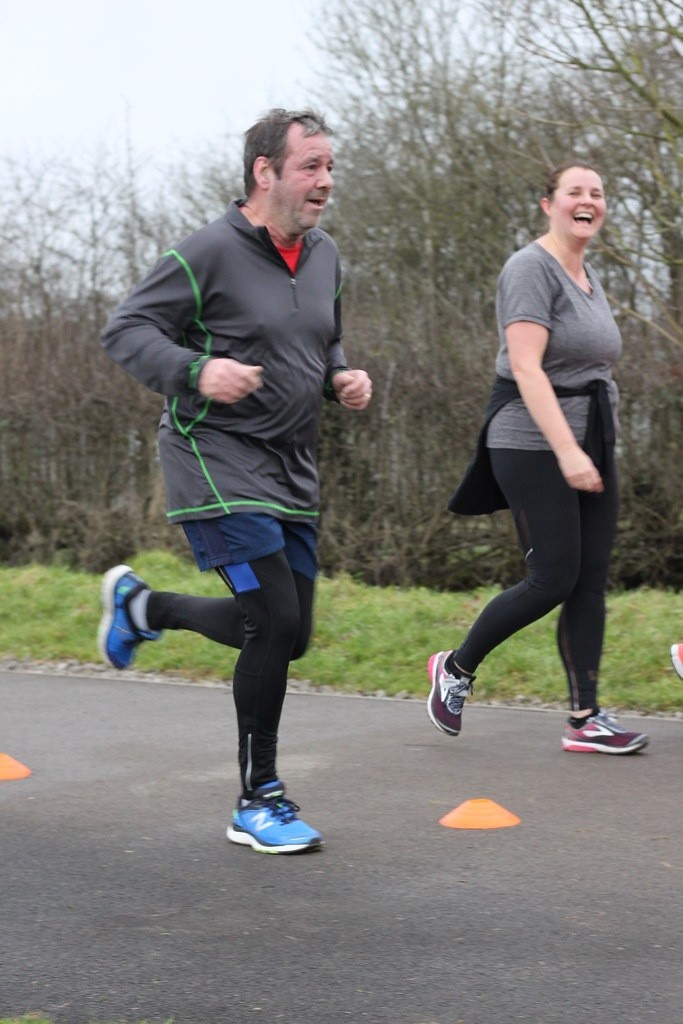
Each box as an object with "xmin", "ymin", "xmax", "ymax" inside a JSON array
[
  {"xmin": 425, "ymin": 160, "xmax": 653, "ymax": 758},
  {"xmin": 93, "ymin": 102, "xmax": 375, "ymax": 860}
]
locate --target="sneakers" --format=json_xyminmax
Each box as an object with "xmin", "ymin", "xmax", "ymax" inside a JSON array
[
  {"xmin": 225, "ymin": 780, "xmax": 324, "ymax": 855},
  {"xmin": 97, "ymin": 563, "xmax": 164, "ymax": 672},
  {"xmin": 561, "ymin": 709, "xmax": 649, "ymax": 755},
  {"xmin": 426, "ymin": 648, "xmax": 476, "ymax": 735}
]
